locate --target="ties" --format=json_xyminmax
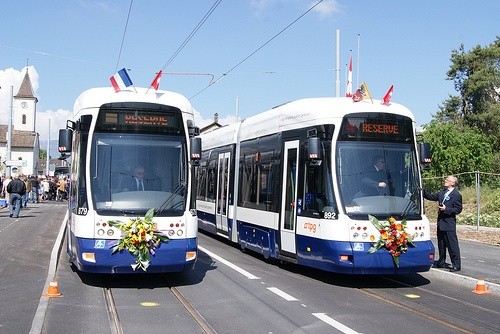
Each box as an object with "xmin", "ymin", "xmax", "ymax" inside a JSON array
[{"xmin": 138, "ymin": 180, "xmax": 143, "ymax": 191}]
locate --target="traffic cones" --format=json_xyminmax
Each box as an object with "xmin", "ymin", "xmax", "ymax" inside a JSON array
[
  {"xmin": 43, "ymin": 280, "xmax": 65, "ymax": 298},
  {"xmin": 472, "ymin": 279, "xmax": 492, "ymax": 295}
]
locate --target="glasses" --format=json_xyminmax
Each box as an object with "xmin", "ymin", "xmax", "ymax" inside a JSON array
[
  {"xmin": 135, "ymin": 171, "xmax": 144, "ymax": 175},
  {"xmin": 445, "ymin": 179, "xmax": 452, "ymax": 182},
  {"xmin": 377, "ymin": 160, "xmax": 386, "ymax": 163}
]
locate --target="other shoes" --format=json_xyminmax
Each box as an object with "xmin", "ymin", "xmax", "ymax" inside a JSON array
[
  {"xmin": 9, "ymin": 213, "xmax": 13, "ymax": 217},
  {"xmin": 450, "ymin": 268, "xmax": 457, "ymax": 272},
  {"xmin": 432, "ymin": 264, "xmax": 445, "ymax": 268},
  {"xmin": 16, "ymin": 215, "xmax": 19, "ymax": 218}
]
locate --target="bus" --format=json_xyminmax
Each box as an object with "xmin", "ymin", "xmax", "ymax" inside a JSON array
[
  {"xmin": 58, "ymin": 86, "xmax": 203, "ymax": 275},
  {"xmin": 193, "ymin": 97, "xmax": 435, "ymax": 275}
]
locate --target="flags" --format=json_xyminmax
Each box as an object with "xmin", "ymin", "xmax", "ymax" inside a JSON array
[
  {"xmin": 382, "ymin": 84, "xmax": 393, "ymax": 105},
  {"xmin": 150, "ymin": 70, "xmax": 162, "ymax": 90},
  {"xmin": 352, "ymin": 82, "xmax": 372, "ymax": 102},
  {"xmin": 346, "ymin": 55, "xmax": 353, "ymax": 97},
  {"xmin": 109, "ymin": 67, "xmax": 134, "ymax": 93}
]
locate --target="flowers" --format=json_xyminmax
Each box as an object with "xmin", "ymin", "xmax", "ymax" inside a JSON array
[
  {"xmin": 372, "ymin": 213, "xmax": 417, "ymax": 268},
  {"xmin": 108, "ymin": 207, "xmax": 168, "ymax": 272}
]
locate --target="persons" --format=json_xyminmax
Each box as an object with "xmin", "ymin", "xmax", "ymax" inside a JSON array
[
  {"xmin": 0, "ymin": 173, "xmax": 70, "ymax": 210},
  {"xmin": 121, "ymin": 164, "xmax": 154, "ymax": 192},
  {"xmin": 6, "ymin": 176, "xmax": 27, "ymax": 218},
  {"xmin": 423, "ymin": 175, "xmax": 463, "ymax": 272},
  {"xmin": 351, "ymin": 154, "xmax": 408, "ymax": 198}
]
